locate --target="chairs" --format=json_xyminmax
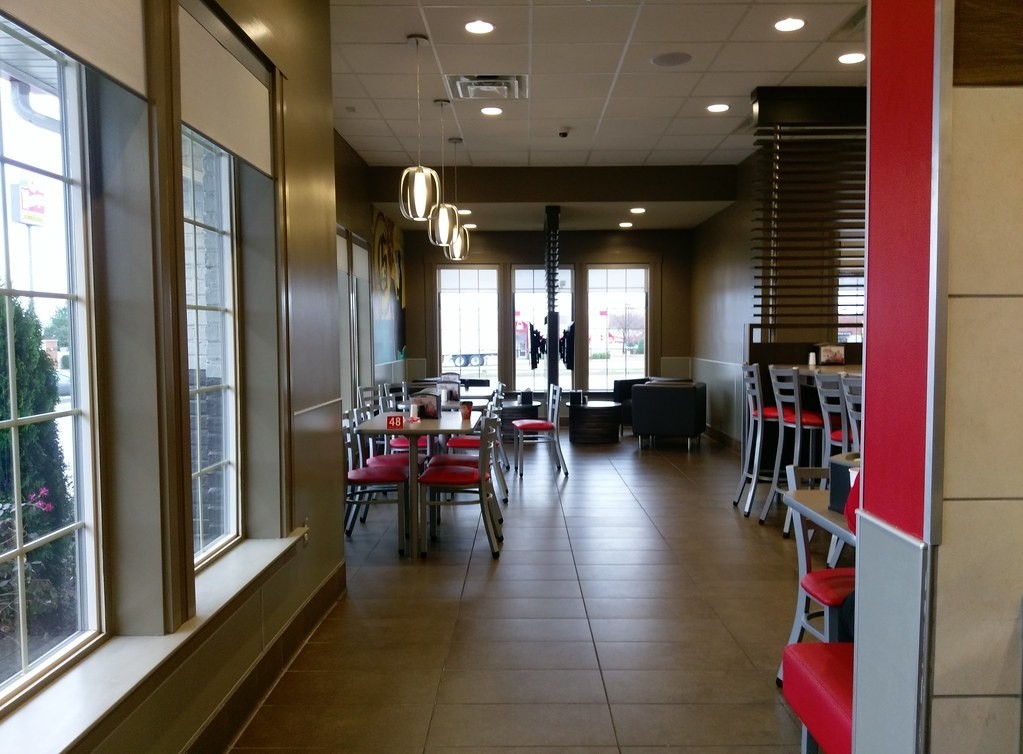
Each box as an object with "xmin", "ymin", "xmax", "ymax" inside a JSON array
[
  {"xmin": 417, "ymin": 415, "xmax": 504, "ymax": 560},
  {"xmin": 354, "ymin": 407, "xmax": 427, "ymax": 526},
  {"xmin": 757, "ymin": 363, "xmax": 841, "ymax": 537},
  {"xmin": 427, "ymin": 409, "xmax": 502, "ymax": 536},
  {"xmin": 512, "ymin": 383, "xmax": 567, "ymax": 478},
  {"xmin": 633, "ymin": 381, "xmax": 707, "ymax": 453},
  {"xmin": 343, "ymin": 410, "xmax": 410, "ymax": 557},
  {"xmin": 733, "ymin": 362, "xmax": 786, "ymax": 519},
  {"xmin": 358, "ymin": 382, "xmax": 440, "ymax": 500},
  {"xmin": 447, "ymin": 382, "xmax": 511, "ymax": 504},
  {"xmin": 805, "ymin": 368, "xmax": 862, "ymax": 568},
  {"xmin": 773, "ymin": 465, "xmax": 853, "ymax": 754}
]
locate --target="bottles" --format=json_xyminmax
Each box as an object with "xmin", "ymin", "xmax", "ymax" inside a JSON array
[
  {"xmin": 583, "ymin": 396, "xmax": 586, "ymax": 404},
  {"xmin": 410, "ymin": 399, "xmax": 418, "ymax": 420},
  {"xmin": 418, "ymin": 400, "xmax": 425, "ymax": 419},
  {"xmin": 809, "ymin": 351, "xmax": 816, "ymax": 364},
  {"xmin": 441, "ymin": 387, "xmax": 453, "ymax": 402}
]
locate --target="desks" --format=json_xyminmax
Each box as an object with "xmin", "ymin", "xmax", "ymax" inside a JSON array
[
  {"xmin": 783, "ymin": 487, "xmax": 857, "ymax": 550},
  {"xmin": 397, "ymin": 398, "xmax": 490, "ymax": 462},
  {"xmin": 354, "ymin": 410, "xmax": 482, "ymax": 562},
  {"xmin": 420, "ymin": 385, "xmax": 497, "ymax": 438},
  {"xmin": 768, "ymin": 365, "xmax": 862, "ymax": 382}
]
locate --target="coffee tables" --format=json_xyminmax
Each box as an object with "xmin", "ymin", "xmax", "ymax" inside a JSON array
[
  {"xmin": 566, "ymin": 401, "xmax": 622, "ymax": 445},
  {"xmin": 502, "ymin": 400, "xmax": 541, "ymax": 444}
]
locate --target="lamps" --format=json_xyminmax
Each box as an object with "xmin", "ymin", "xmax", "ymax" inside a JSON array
[
  {"xmin": 445, "ymin": 137, "xmax": 469, "ymax": 261},
  {"xmin": 397, "ymin": 33, "xmax": 441, "ymax": 222},
  {"xmin": 428, "ymin": 99, "xmax": 459, "ymax": 247}
]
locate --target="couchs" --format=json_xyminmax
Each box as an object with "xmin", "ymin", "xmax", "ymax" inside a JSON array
[{"xmin": 614, "ymin": 376, "xmax": 694, "ymax": 429}]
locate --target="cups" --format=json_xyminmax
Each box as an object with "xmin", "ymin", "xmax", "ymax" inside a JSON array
[{"xmin": 459, "ymin": 401, "xmax": 473, "ymax": 419}]
